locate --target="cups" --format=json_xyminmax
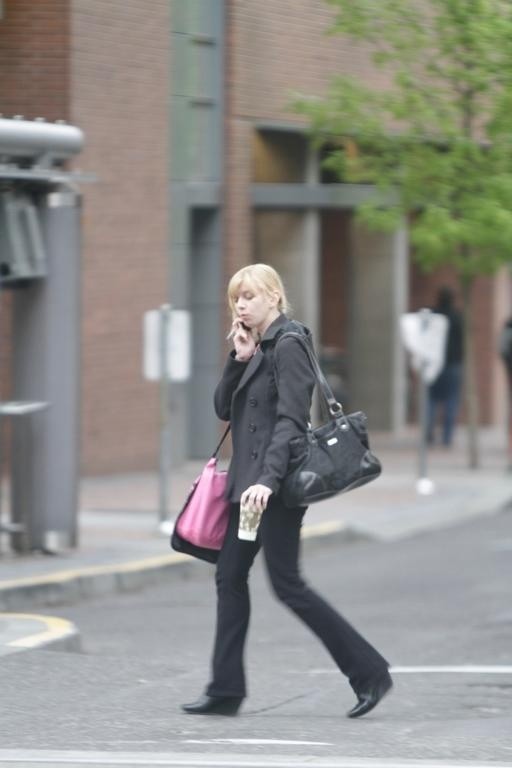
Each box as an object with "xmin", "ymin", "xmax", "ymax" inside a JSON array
[{"xmin": 237, "ymin": 499, "xmax": 265, "ymax": 541}]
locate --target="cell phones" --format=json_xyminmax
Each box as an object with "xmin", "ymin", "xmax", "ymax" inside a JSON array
[{"xmin": 238, "ymin": 322, "xmax": 251, "ymax": 331}]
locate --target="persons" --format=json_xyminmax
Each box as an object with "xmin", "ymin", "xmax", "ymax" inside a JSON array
[
  {"xmin": 418, "ymin": 285, "xmax": 469, "ymax": 446},
  {"xmin": 180, "ymin": 264, "xmax": 395, "ymax": 720}
]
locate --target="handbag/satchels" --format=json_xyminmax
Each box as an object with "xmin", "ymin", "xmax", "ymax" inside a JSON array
[
  {"xmin": 171, "ymin": 456, "xmax": 233, "ymax": 563},
  {"xmin": 282, "ymin": 405, "xmax": 383, "ymax": 507}
]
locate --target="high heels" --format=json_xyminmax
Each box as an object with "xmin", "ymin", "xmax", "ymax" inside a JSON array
[
  {"xmin": 345, "ymin": 668, "xmax": 395, "ymax": 719},
  {"xmin": 179, "ymin": 693, "xmax": 244, "ymax": 718}
]
